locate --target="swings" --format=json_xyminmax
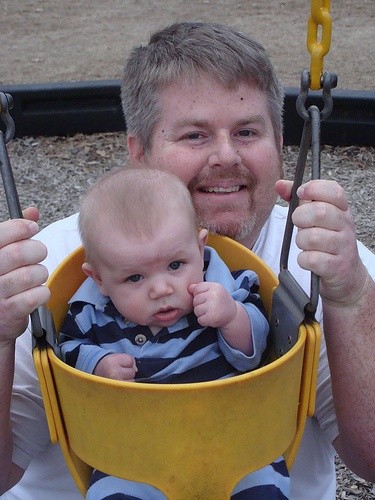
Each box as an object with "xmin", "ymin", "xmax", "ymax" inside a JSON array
[{"xmin": 0, "ymin": 0, "xmax": 339, "ymax": 500}]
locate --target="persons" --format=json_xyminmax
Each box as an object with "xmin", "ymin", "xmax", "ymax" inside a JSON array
[
  {"xmin": 0, "ymin": 21, "xmax": 375, "ymax": 500},
  {"xmin": 56, "ymin": 166, "xmax": 291, "ymax": 500}
]
